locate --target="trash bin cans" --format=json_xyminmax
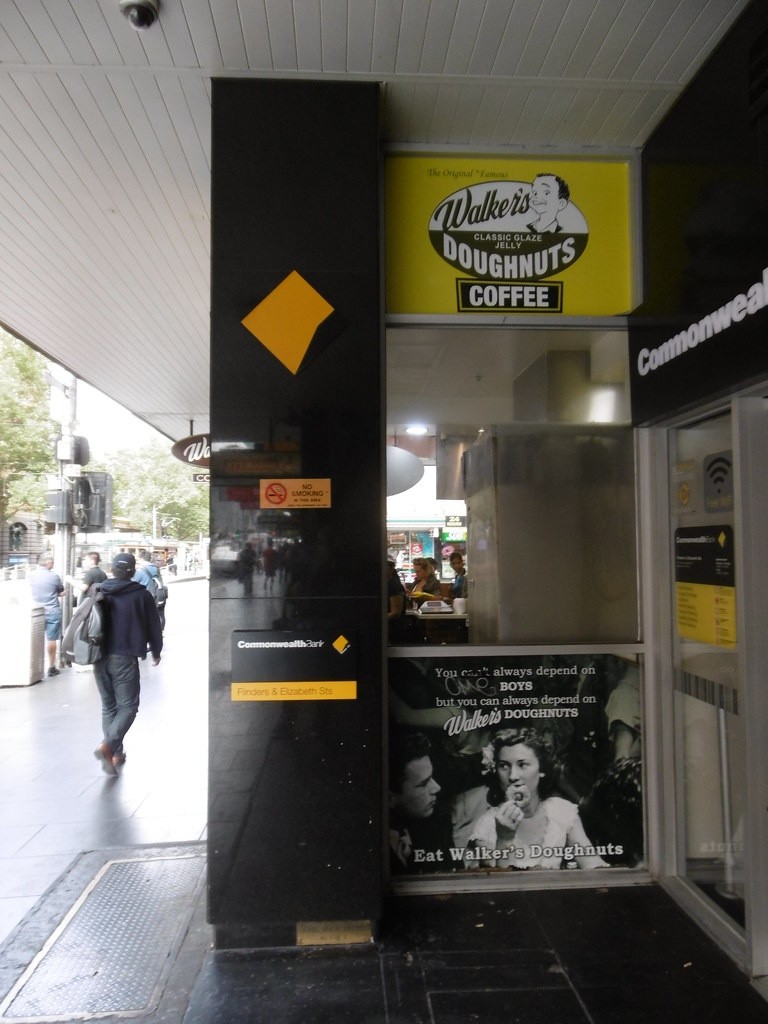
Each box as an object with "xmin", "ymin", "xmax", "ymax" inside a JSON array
[{"xmin": 1, "ymin": 604, "xmax": 46, "ymax": 688}]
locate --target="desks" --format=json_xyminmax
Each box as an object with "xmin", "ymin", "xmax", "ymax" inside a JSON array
[{"xmin": 405, "ymin": 609, "xmax": 469, "ymax": 645}]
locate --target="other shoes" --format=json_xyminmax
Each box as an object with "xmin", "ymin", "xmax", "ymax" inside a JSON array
[
  {"xmin": 113, "ymin": 751, "xmax": 126, "ymax": 765},
  {"xmin": 48, "ymin": 666, "xmax": 60, "ymax": 677},
  {"xmin": 93, "ymin": 743, "xmax": 118, "ymax": 776}
]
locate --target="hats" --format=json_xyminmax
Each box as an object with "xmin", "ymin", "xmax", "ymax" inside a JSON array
[{"xmin": 112, "ymin": 553, "xmax": 136, "ymax": 571}]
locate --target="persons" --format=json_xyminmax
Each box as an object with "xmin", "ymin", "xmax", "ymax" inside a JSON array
[
  {"xmin": 32, "ymin": 545, "xmax": 166, "ymax": 773},
  {"xmin": 385, "ymin": 662, "xmax": 644, "ymax": 877},
  {"xmin": 236, "ymin": 538, "xmax": 293, "ymax": 597},
  {"xmin": 386, "ymin": 551, "xmax": 468, "ymax": 644}
]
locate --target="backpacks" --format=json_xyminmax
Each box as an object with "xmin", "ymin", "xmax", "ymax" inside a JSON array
[
  {"xmin": 140, "ymin": 566, "xmax": 168, "ymax": 611},
  {"xmin": 61, "ymin": 586, "xmax": 105, "ymax": 666}
]
